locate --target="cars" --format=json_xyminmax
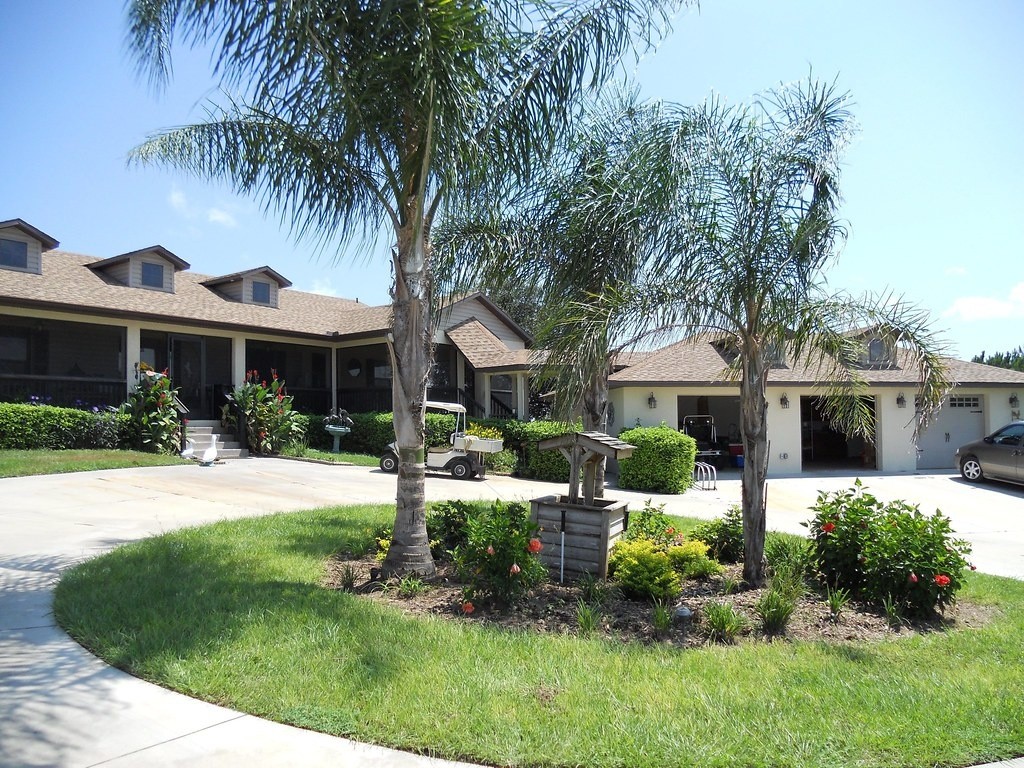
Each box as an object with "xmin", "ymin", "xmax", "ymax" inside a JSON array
[{"xmin": 954, "ymin": 420, "xmax": 1024, "ymax": 486}]
